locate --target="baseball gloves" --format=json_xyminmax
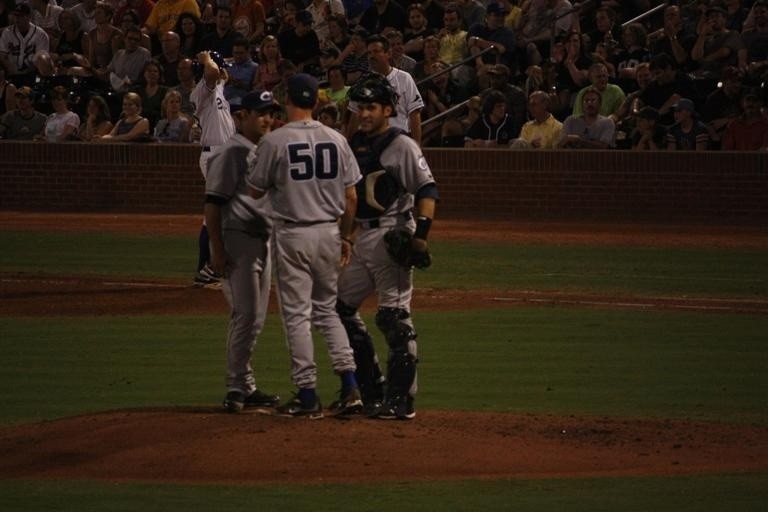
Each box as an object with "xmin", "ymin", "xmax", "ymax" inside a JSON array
[{"xmin": 383, "ymin": 224, "xmax": 433, "ymax": 270}]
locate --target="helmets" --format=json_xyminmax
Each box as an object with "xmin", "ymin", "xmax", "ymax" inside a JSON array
[
  {"xmin": 191, "ymin": 51, "xmax": 232, "ymax": 73},
  {"xmin": 347, "ymin": 70, "xmax": 401, "ymax": 118}
]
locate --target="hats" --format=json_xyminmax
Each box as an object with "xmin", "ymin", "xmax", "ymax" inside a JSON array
[
  {"xmin": 487, "ymin": 3, "xmax": 504, "ymax": 14},
  {"xmin": 705, "ymin": 6, "xmax": 725, "ymax": 16},
  {"xmin": 743, "ymin": 87, "xmax": 759, "ymax": 99},
  {"xmin": 15, "ymin": 87, "xmax": 32, "ymax": 98},
  {"xmin": 9, "ymin": 4, "xmax": 29, "ymax": 17},
  {"xmin": 633, "ymin": 107, "xmax": 660, "ymax": 119},
  {"xmin": 673, "ymin": 98, "xmax": 694, "ymax": 111},
  {"xmin": 296, "ymin": 10, "xmax": 315, "ymax": 26},
  {"xmin": 288, "ymin": 75, "xmax": 318, "ymax": 107},
  {"xmin": 488, "ymin": 63, "xmax": 507, "ymax": 76},
  {"xmin": 726, "ymin": 66, "xmax": 741, "ymax": 80},
  {"xmin": 241, "ymin": 90, "xmax": 281, "ymax": 113},
  {"xmin": 319, "ymin": 48, "xmax": 339, "ymax": 59}
]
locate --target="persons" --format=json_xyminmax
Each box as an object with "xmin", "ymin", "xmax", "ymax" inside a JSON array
[
  {"xmin": 335, "ymin": 75, "xmax": 434, "ymax": 421},
  {"xmin": 202, "ymin": 91, "xmax": 281, "ymax": 410},
  {"xmin": 192, "ymin": 52, "xmax": 244, "ymax": 284},
  {"xmin": 245, "ymin": 78, "xmax": 361, "ymax": 420},
  {"xmin": 0, "ymin": 2, "xmax": 768, "ymax": 153}
]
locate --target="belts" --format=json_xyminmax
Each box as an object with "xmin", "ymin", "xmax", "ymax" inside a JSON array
[{"xmin": 361, "ymin": 213, "xmax": 409, "ymax": 228}]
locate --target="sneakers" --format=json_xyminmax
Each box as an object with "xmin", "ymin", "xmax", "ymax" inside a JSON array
[
  {"xmin": 329, "ymin": 384, "xmax": 415, "ymax": 421},
  {"xmin": 223, "ymin": 391, "xmax": 245, "ymax": 412},
  {"xmin": 193, "ymin": 264, "xmax": 221, "ymax": 286},
  {"xmin": 246, "ymin": 390, "xmax": 277, "ymax": 406},
  {"xmin": 276, "ymin": 394, "xmax": 320, "ymax": 415}
]
